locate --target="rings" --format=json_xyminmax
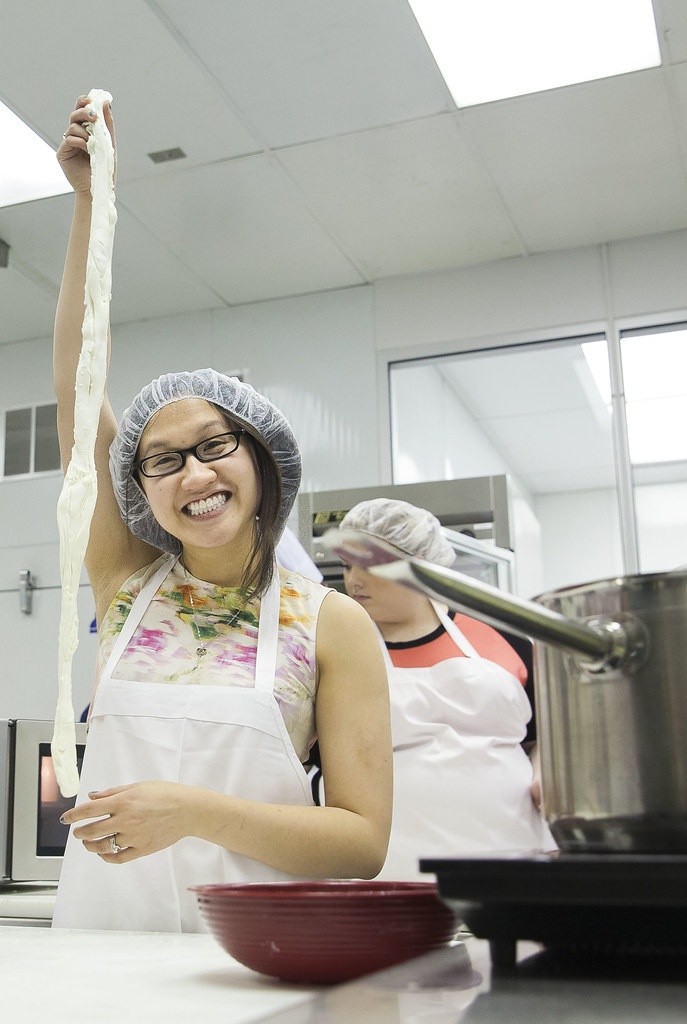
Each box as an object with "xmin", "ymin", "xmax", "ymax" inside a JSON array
[{"xmin": 109, "ymin": 834, "xmax": 128, "ymax": 854}]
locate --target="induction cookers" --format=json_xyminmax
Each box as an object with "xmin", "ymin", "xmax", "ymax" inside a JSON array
[{"xmin": 417, "ymin": 847, "xmax": 687, "ymax": 984}]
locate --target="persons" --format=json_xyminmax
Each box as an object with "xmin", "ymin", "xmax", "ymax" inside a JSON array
[
  {"xmin": 54, "ymin": 93, "xmax": 392, "ymax": 930},
  {"xmin": 340, "ymin": 498, "xmax": 559, "ymax": 933}
]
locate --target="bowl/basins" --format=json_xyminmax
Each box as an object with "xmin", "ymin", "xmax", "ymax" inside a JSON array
[{"xmin": 188, "ymin": 881, "xmax": 465, "ymax": 986}]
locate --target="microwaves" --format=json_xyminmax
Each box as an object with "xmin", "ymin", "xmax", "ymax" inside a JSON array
[{"xmin": 0, "ymin": 717, "xmax": 88, "ymax": 884}]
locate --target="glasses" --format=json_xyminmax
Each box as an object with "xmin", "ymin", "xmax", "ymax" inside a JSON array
[{"xmin": 133, "ymin": 430, "xmax": 248, "ymax": 478}]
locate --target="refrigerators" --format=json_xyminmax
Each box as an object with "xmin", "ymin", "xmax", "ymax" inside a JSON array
[{"xmin": 297, "ymin": 473, "xmax": 543, "ymax": 598}]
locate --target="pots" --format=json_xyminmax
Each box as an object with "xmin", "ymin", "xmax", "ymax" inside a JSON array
[{"xmin": 318, "ymin": 523, "xmax": 686, "ymax": 857}]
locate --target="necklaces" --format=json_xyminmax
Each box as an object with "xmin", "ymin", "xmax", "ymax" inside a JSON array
[{"xmin": 182, "ymin": 556, "xmax": 246, "ymax": 655}]
